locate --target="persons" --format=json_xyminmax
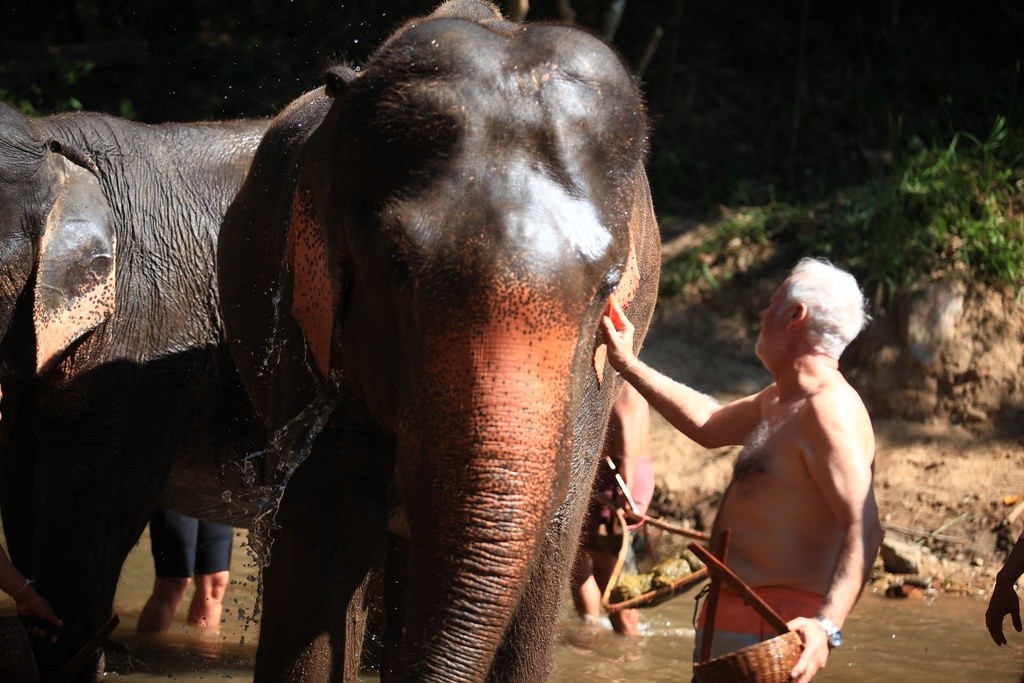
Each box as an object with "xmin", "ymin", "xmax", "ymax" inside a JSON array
[
  {"xmin": 0, "ymin": 548, "xmax": 62, "ymax": 642},
  {"xmin": 987, "ymin": 527, "xmax": 1023, "ymax": 646},
  {"xmin": 572, "ymin": 377, "xmax": 651, "ymax": 636},
  {"xmin": 602, "ymin": 253, "xmax": 884, "ymax": 683},
  {"xmin": 134, "ymin": 506, "xmax": 231, "ymax": 630}
]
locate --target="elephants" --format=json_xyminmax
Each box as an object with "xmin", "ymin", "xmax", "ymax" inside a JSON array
[{"xmin": 0, "ymin": 1, "xmax": 661, "ymax": 683}]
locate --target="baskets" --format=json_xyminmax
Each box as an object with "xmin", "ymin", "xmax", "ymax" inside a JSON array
[{"xmin": 687, "ymin": 528, "xmax": 802, "ymax": 683}]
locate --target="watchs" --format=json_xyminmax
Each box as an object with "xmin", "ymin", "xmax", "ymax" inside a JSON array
[{"xmin": 816, "ymin": 613, "xmax": 841, "ymax": 648}]
